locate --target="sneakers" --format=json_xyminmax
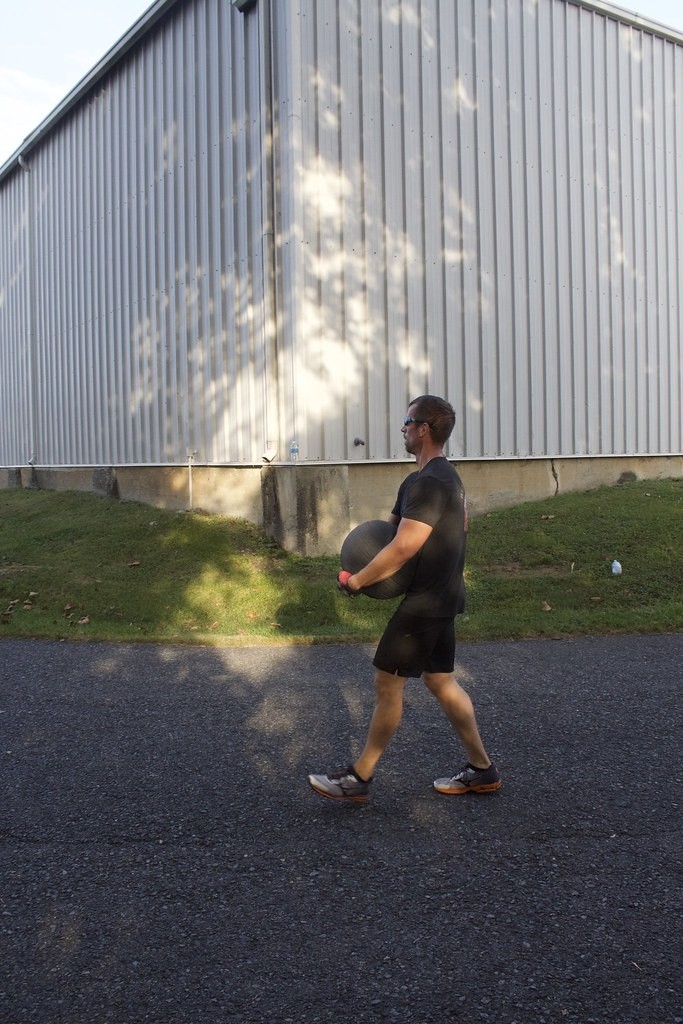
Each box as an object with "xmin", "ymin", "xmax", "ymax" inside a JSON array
[
  {"xmin": 433, "ymin": 762, "xmax": 502, "ymax": 794},
  {"xmin": 307, "ymin": 767, "xmax": 374, "ymax": 803}
]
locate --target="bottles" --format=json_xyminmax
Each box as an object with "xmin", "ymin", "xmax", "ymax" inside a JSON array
[{"xmin": 612, "ymin": 559, "xmax": 622, "ymax": 574}]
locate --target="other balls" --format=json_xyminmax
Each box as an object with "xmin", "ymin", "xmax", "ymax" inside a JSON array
[{"xmin": 339, "ymin": 519, "xmax": 421, "ymax": 601}]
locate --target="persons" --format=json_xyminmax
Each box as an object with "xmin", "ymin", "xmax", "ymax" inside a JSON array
[{"xmin": 307, "ymin": 395, "xmax": 502, "ymax": 803}]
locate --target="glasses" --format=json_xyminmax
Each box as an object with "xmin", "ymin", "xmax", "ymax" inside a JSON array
[{"xmin": 404, "ymin": 417, "xmax": 436, "ymax": 432}]
